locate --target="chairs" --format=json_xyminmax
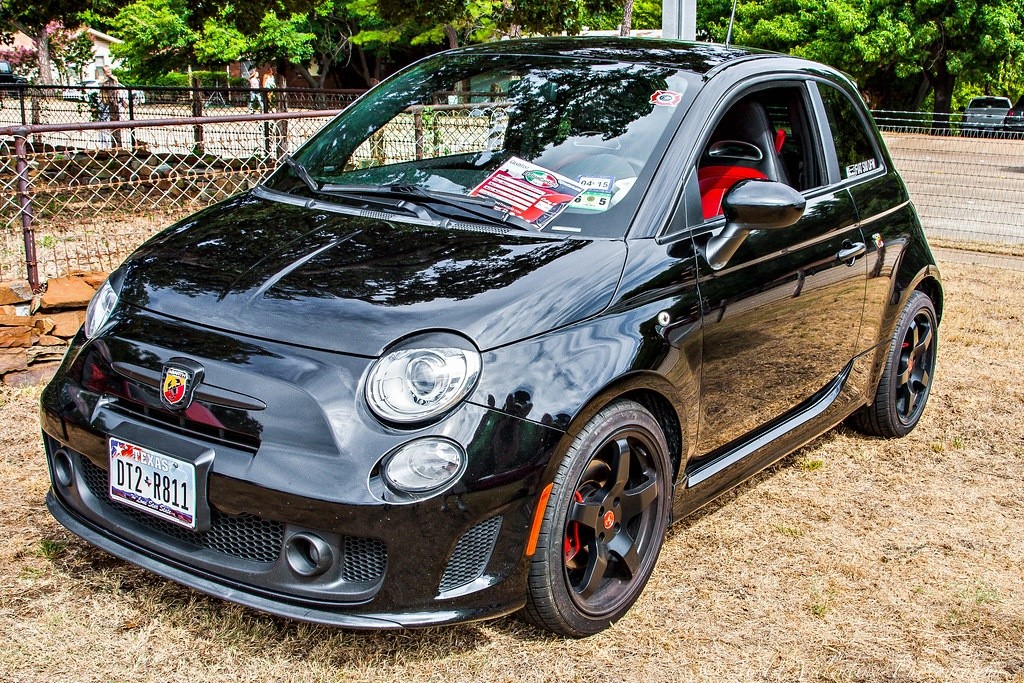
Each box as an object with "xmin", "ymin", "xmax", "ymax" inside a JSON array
[
  {"xmin": 698, "ymin": 101, "xmax": 791, "ymax": 220},
  {"xmin": 540, "ymin": 103, "xmax": 636, "ymax": 172}
]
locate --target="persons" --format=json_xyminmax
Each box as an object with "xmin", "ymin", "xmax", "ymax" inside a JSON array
[
  {"xmin": 99, "ymin": 65, "xmax": 123, "ymax": 148},
  {"xmin": 249, "ymin": 61, "xmax": 277, "ymax": 114}
]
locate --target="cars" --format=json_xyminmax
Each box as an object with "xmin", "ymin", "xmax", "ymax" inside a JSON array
[
  {"xmin": 63, "ymin": 79, "xmax": 145, "ymax": 106},
  {"xmin": 958, "ymin": 96, "xmax": 1014, "ymax": 138},
  {"xmin": 0, "ymin": 61, "xmax": 29, "ymax": 97},
  {"xmin": 38, "ymin": 35, "xmax": 944, "ymax": 641},
  {"xmin": 1003, "ymin": 94, "xmax": 1024, "ymax": 139}
]
